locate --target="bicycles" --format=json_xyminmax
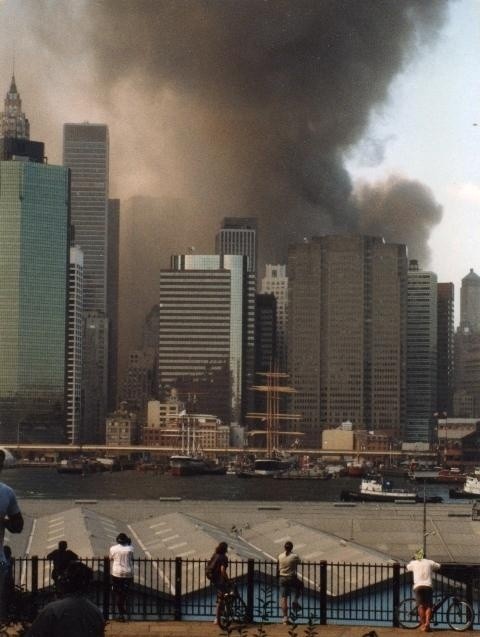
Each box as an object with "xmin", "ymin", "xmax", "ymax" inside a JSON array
[
  {"xmin": 216, "ymin": 579, "xmax": 248, "ymax": 631},
  {"xmin": 394, "ymin": 587, "xmax": 474, "ymax": 631}
]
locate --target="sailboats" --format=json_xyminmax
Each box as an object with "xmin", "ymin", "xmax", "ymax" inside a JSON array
[{"xmin": 169, "ymin": 392, "xmax": 224, "ymax": 478}]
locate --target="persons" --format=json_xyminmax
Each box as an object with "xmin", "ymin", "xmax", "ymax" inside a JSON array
[
  {"xmin": 48, "ymin": 541, "xmax": 79, "ymax": 585},
  {"xmin": 107, "ymin": 532, "xmax": 134, "ymax": 622},
  {"xmin": 1, "ymin": 546, "xmax": 18, "ymax": 618},
  {"xmin": 275, "ymin": 541, "xmax": 303, "ymax": 625},
  {"xmin": 25, "ymin": 575, "xmax": 105, "ymax": 637},
  {"xmin": 1, "ymin": 449, "xmax": 24, "ymax": 564},
  {"xmin": 406, "ymin": 552, "xmax": 441, "ymax": 631},
  {"xmin": 206, "ymin": 541, "xmax": 234, "ymax": 624}
]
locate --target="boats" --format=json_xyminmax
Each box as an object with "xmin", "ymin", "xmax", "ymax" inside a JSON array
[{"xmin": 224, "ymin": 441, "xmax": 480, "ymax": 502}]
